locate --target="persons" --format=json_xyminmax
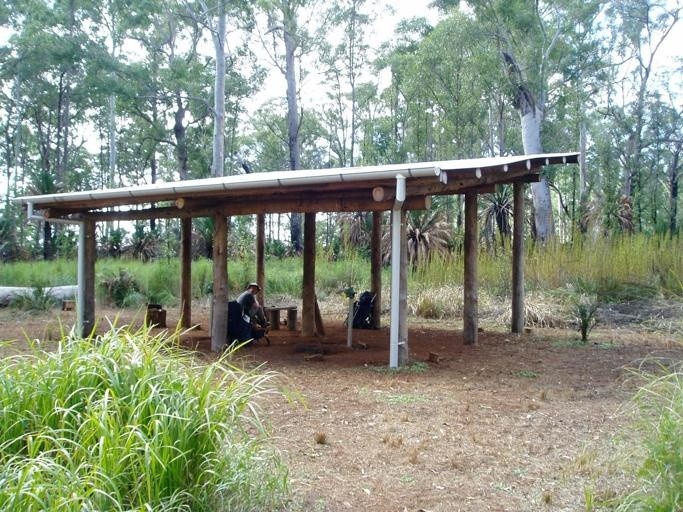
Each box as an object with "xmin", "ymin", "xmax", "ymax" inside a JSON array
[{"xmin": 235, "ymin": 282, "xmax": 271, "ymax": 329}]
